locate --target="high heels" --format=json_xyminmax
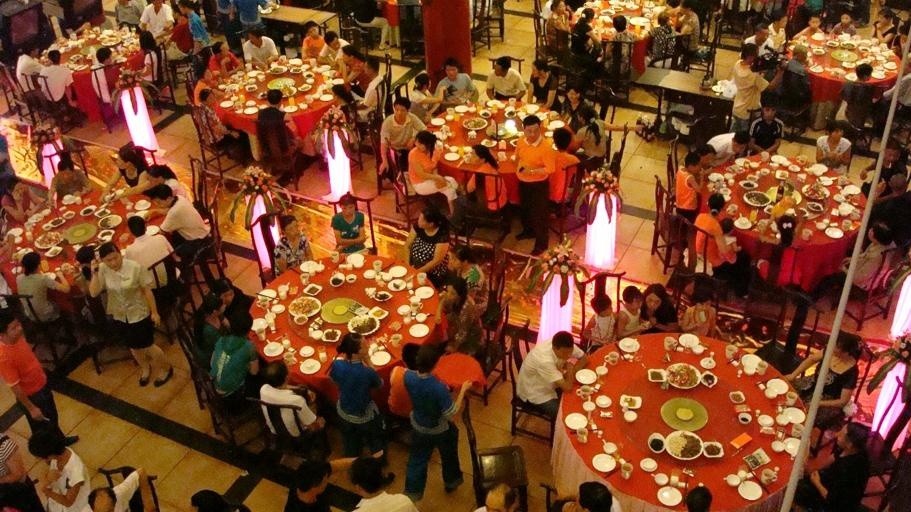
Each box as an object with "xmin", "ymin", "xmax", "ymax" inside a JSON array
[
  {"xmin": 153, "ymin": 364, "xmax": 173, "ymax": 387},
  {"xmin": 138, "ymin": 363, "xmax": 152, "ymax": 386}
]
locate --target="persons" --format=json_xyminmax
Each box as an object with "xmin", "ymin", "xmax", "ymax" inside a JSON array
[{"xmin": 2, "ymin": 1, "xmax": 911, "ymax": 512}]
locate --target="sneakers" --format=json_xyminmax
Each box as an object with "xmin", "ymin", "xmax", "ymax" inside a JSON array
[
  {"xmin": 63, "ymin": 435, "xmax": 80, "ymax": 447},
  {"xmin": 382, "ymin": 471, "xmax": 396, "ymax": 485},
  {"xmin": 515, "ymin": 230, "xmax": 535, "ymax": 241},
  {"xmin": 530, "ymin": 247, "xmax": 546, "ymax": 256}
]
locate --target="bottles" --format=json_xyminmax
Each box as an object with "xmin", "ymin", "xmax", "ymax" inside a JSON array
[{"xmin": 702, "ymin": 75, "xmax": 712, "ymax": 91}]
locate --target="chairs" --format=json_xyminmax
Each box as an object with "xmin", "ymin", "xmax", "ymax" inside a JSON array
[
  {"xmin": 1, "ymin": 13, "xmax": 176, "ymax": 134},
  {"xmin": 175, "ymin": 189, "xmax": 512, "ymax": 461},
  {"xmin": 530, "ymin": 0, "xmax": 724, "ymax": 98},
  {"xmin": 366, "ymin": 53, "xmax": 630, "ymax": 248},
  {"xmin": 460, "ymin": 394, "xmax": 530, "ymax": 510},
  {"xmin": 179, "ymin": 0, "xmax": 396, "ymax": 190},
  {"xmin": 97, "ymin": 465, "xmax": 162, "ymax": 512},
  {"xmin": 350, "ymin": 0, "xmax": 507, "ymax": 60},
  {"xmin": 0, "ymin": 140, "xmax": 228, "ymax": 375},
  {"xmin": 720, "ymin": 1, "xmax": 847, "ymax": 36},
  {"xmin": 651, "ymin": 108, "xmax": 911, "ymax": 333},
  {"xmin": 508, "ymin": 269, "xmax": 911, "ymax": 510},
  {"xmin": 744, "ymin": 16, "xmax": 911, "ymax": 156}
]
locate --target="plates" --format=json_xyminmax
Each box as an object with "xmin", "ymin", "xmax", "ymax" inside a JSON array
[
  {"xmin": 618, "ymin": 338, "xmax": 640, "ymax": 352},
  {"xmin": 219, "ymin": 58, "xmax": 345, "ymax": 114},
  {"xmin": 790, "ymin": 32, "xmax": 898, "ymax": 83},
  {"xmin": 566, "ymin": 412, "xmax": 588, "ymax": 429},
  {"xmin": 46, "ymin": 25, "xmax": 141, "ymax": 70},
  {"xmin": 431, "ymin": 98, "xmax": 567, "ymax": 166},
  {"xmin": 587, "ymin": 345, "xmax": 783, "ymax": 487},
  {"xmin": 658, "ymin": 487, "xmax": 683, "ymax": 506},
  {"xmin": 250, "ymin": 251, "xmax": 435, "ymax": 374},
  {"xmin": 577, "ymin": 0, "xmax": 662, "ymax": 36},
  {"xmin": 575, "ymin": 369, "xmax": 597, "ymax": 384},
  {"xmin": 742, "ymin": 354, "xmax": 762, "ymax": 367},
  {"xmin": 712, "ymin": 80, "xmax": 730, "ymax": 93},
  {"xmin": 767, "ymin": 378, "xmax": 789, "ymax": 394},
  {"xmin": 261, "ymin": 10, "xmax": 273, "ymax": 15},
  {"xmin": 738, "ymin": 481, "xmax": 763, "ymax": 500},
  {"xmin": 708, "ymin": 151, "xmax": 863, "ymax": 239},
  {"xmin": 8, "ymin": 190, "xmax": 159, "ymax": 281},
  {"xmin": 679, "ymin": 334, "xmax": 700, "ymax": 348},
  {"xmin": 592, "ymin": 453, "xmax": 617, "ymax": 472},
  {"xmin": 784, "ymin": 406, "xmax": 806, "ymax": 423},
  {"xmin": 784, "ymin": 437, "xmax": 800, "ymax": 457}
]
